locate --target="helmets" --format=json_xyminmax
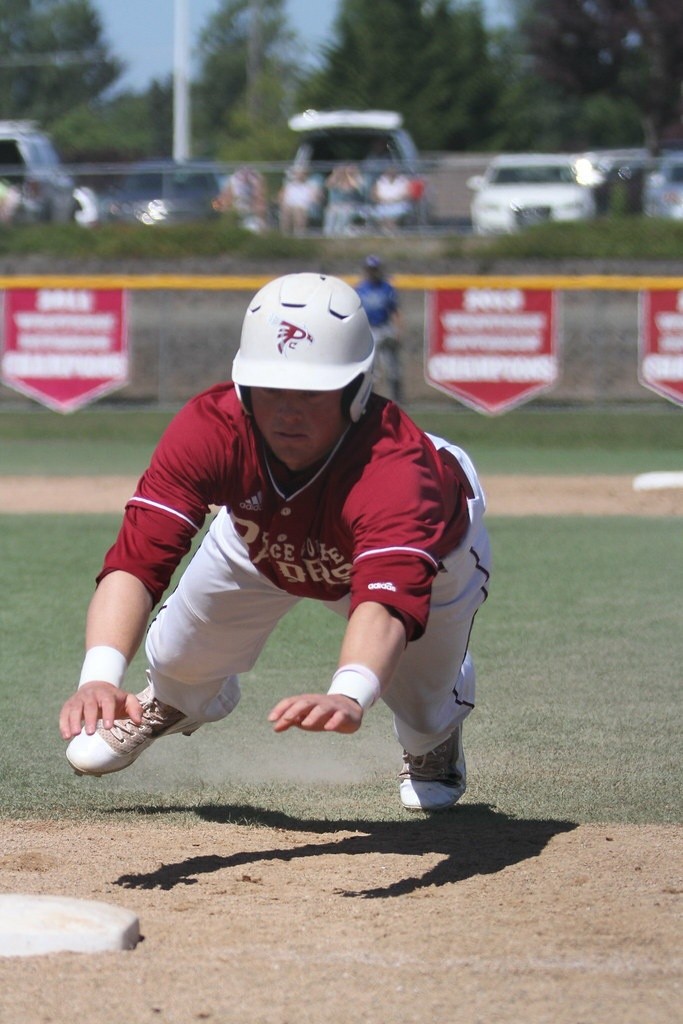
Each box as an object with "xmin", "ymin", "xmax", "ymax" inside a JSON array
[{"xmin": 230, "ymin": 272, "xmax": 377, "ymax": 431}]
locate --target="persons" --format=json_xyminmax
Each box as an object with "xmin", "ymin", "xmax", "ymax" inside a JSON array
[
  {"xmin": 59, "ymin": 273, "xmax": 488, "ymax": 810},
  {"xmin": 370, "ymin": 170, "xmax": 415, "ymax": 237},
  {"xmin": 324, "ymin": 169, "xmax": 366, "ymax": 235},
  {"xmin": 354, "ymin": 255, "xmax": 400, "ymax": 402},
  {"xmin": 221, "ymin": 169, "xmax": 266, "ymax": 234},
  {"xmin": 274, "ymin": 171, "xmax": 323, "ymax": 236}
]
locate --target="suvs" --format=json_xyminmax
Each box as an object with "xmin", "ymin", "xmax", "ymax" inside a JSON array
[
  {"xmin": 277, "ymin": 108, "xmax": 435, "ymax": 241},
  {"xmin": 0, "ymin": 119, "xmax": 77, "ymax": 232}
]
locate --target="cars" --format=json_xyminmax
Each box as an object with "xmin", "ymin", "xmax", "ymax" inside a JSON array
[
  {"xmin": 644, "ymin": 153, "xmax": 683, "ymax": 227},
  {"xmin": 465, "ymin": 152, "xmax": 594, "ymax": 231},
  {"xmin": 94, "ymin": 162, "xmax": 244, "ymax": 228}
]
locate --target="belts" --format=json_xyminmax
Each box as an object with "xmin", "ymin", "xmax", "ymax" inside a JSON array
[{"xmin": 437, "ymin": 446, "xmax": 477, "ymax": 572}]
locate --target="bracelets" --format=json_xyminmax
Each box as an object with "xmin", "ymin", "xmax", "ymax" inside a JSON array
[{"xmin": 333, "ymin": 666, "xmax": 377, "ymax": 698}]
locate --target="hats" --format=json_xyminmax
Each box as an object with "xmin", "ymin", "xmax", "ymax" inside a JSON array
[{"xmin": 365, "ymin": 255, "xmax": 380, "ymax": 268}]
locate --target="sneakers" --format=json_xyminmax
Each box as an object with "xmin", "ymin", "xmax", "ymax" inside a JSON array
[
  {"xmin": 399, "ymin": 720, "xmax": 466, "ymax": 810},
  {"xmin": 66, "ymin": 669, "xmax": 205, "ymax": 777}
]
